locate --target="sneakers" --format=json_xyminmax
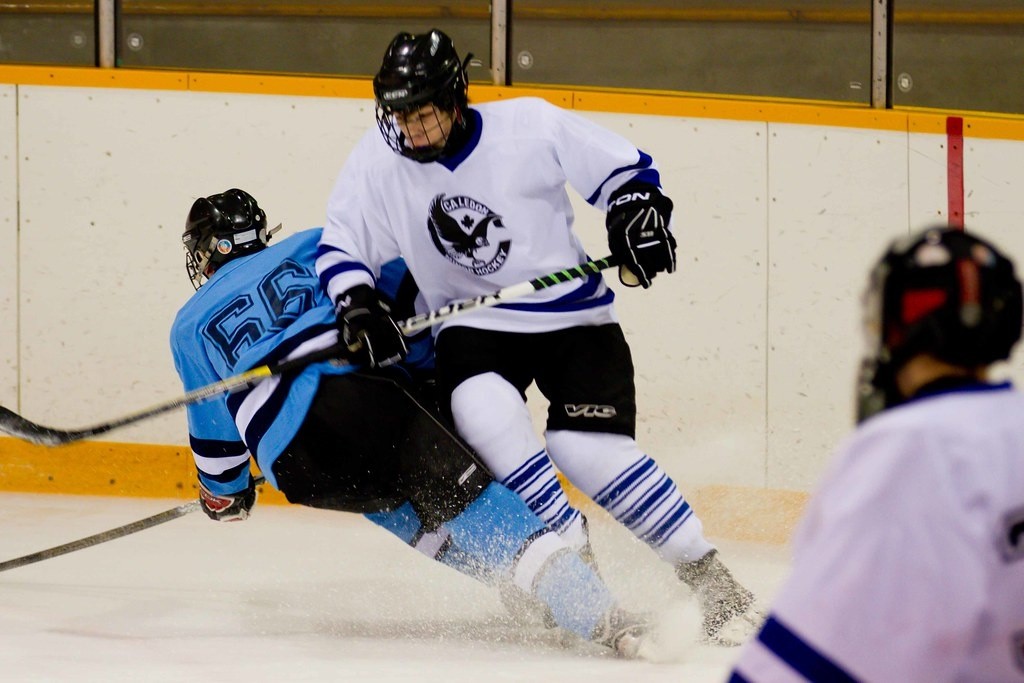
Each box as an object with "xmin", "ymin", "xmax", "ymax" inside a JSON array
[
  {"xmin": 590, "ymin": 603, "xmax": 660, "ymax": 660},
  {"xmin": 676, "ymin": 548, "xmax": 766, "ymax": 647},
  {"xmin": 587, "ymin": 595, "xmax": 668, "ymax": 659},
  {"xmin": 543, "ymin": 513, "xmax": 604, "ymax": 629}
]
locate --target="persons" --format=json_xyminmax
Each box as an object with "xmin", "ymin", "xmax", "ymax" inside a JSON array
[
  {"xmin": 170, "ymin": 189, "xmax": 666, "ymax": 661},
  {"xmin": 724, "ymin": 224, "xmax": 1024, "ymax": 683},
  {"xmin": 315, "ymin": 26, "xmax": 766, "ymax": 646}
]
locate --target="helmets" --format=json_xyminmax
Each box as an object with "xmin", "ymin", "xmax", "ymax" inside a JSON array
[
  {"xmin": 181, "ymin": 188, "xmax": 267, "ymax": 292},
  {"xmin": 851, "ymin": 224, "xmax": 1024, "ymax": 425},
  {"xmin": 372, "ymin": 28, "xmax": 474, "ymax": 164}
]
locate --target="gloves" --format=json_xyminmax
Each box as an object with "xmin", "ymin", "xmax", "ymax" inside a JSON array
[
  {"xmin": 332, "ymin": 284, "xmax": 408, "ymax": 367},
  {"xmin": 196, "ymin": 470, "xmax": 257, "ymax": 522},
  {"xmin": 605, "ymin": 179, "xmax": 677, "ymax": 289}
]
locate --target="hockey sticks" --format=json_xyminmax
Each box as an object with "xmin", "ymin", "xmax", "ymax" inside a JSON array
[
  {"xmin": 0, "ymin": 255, "xmax": 623, "ymax": 448},
  {"xmin": 0, "ymin": 473, "xmax": 268, "ymax": 573}
]
